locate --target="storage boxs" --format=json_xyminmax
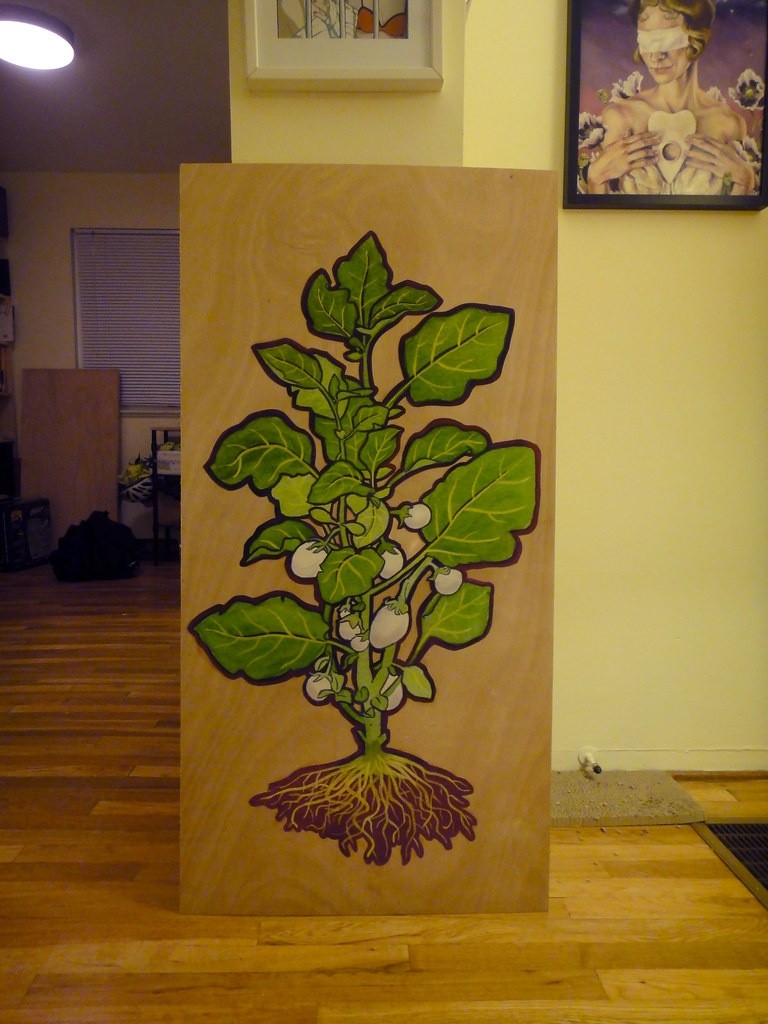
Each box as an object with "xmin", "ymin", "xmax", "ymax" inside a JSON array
[
  {"xmin": 1, "ymin": 496, "xmax": 51, "ymax": 570},
  {"xmin": 157, "ymin": 450, "xmax": 180, "ymax": 475}
]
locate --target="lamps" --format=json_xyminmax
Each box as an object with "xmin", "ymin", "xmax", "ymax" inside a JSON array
[{"xmin": 0, "ymin": 2, "xmax": 76, "ymax": 71}]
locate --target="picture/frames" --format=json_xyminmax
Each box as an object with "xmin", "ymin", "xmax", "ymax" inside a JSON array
[
  {"xmin": 244, "ymin": 0, "xmax": 443, "ymax": 95},
  {"xmin": 562, "ymin": 0, "xmax": 768, "ymax": 213}
]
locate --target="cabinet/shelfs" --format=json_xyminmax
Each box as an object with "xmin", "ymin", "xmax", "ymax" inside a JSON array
[
  {"xmin": 152, "ymin": 426, "xmax": 181, "ymax": 566},
  {"xmin": 1, "ymin": 259, "xmax": 15, "ymax": 504}
]
